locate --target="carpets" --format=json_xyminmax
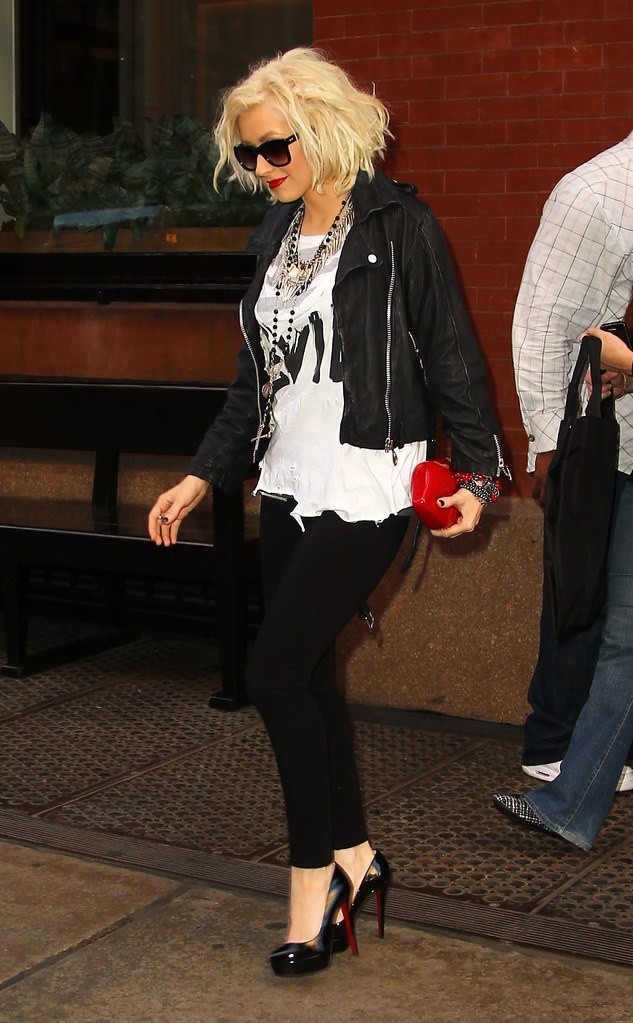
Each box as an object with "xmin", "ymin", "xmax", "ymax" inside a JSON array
[{"xmin": 0, "ymin": 631, "xmax": 633, "ymax": 969}]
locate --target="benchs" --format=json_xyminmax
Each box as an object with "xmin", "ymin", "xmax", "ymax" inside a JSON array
[{"xmin": 0, "ymin": 250, "xmax": 269, "ymax": 709}]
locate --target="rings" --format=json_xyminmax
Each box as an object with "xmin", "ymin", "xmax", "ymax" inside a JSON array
[
  {"xmin": 586, "ymin": 393, "xmax": 589, "ymax": 402},
  {"xmin": 158, "ymin": 516, "xmax": 163, "ymax": 519}
]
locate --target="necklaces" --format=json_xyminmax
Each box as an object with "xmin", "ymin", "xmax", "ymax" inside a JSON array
[{"xmin": 252, "ymin": 188, "xmax": 354, "ymax": 442}]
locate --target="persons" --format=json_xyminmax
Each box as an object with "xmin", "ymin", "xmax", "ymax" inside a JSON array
[
  {"xmin": 511, "ymin": 129, "xmax": 633, "ymax": 792},
  {"xmin": 493, "ymin": 284, "xmax": 632, "ymax": 852},
  {"xmin": 146, "ymin": 46, "xmax": 515, "ymax": 978}
]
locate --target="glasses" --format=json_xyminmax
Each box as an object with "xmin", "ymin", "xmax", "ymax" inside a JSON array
[{"xmin": 232, "ymin": 124, "xmax": 316, "ymax": 172}]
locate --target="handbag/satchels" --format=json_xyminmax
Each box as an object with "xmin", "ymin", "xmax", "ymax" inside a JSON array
[{"xmin": 542, "ymin": 336, "xmax": 621, "ymax": 642}]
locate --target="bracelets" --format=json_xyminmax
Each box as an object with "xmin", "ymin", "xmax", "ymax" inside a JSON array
[
  {"xmin": 458, "ymin": 473, "xmax": 502, "ymax": 509},
  {"xmin": 620, "ymin": 372, "xmax": 626, "ymax": 396}
]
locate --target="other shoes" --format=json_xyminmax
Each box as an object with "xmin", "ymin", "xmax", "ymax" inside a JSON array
[
  {"xmin": 492, "ymin": 793, "xmax": 574, "ymax": 845},
  {"xmin": 520, "ymin": 759, "xmax": 633, "ymax": 793}
]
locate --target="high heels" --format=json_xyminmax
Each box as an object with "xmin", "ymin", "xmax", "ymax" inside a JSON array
[{"xmin": 268, "ymin": 848, "xmax": 391, "ymax": 976}]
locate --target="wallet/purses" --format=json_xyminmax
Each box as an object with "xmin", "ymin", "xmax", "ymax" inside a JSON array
[{"xmin": 410, "ymin": 458, "xmax": 463, "ymax": 531}]
